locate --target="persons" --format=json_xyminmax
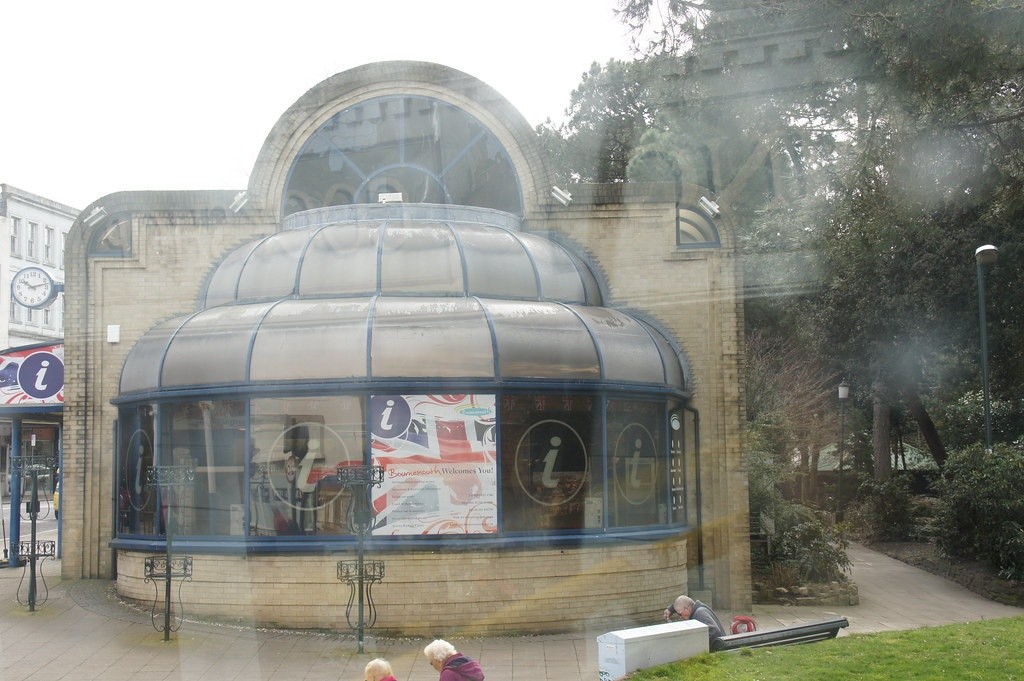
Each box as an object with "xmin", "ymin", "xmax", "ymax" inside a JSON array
[
  {"xmin": 663, "ymin": 595, "xmax": 726, "ymax": 643},
  {"xmin": 424, "ymin": 639, "xmax": 486, "ymax": 681},
  {"xmin": 365, "ymin": 658, "xmax": 399, "ymax": 681}
]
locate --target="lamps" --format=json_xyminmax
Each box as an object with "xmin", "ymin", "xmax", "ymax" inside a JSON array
[
  {"xmin": 549, "ymin": 185, "xmax": 574, "ymax": 207},
  {"xmin": 378, "ymin": 191, "xmax": 403, "ymax": 204},
  {"xmin": 697, "ymin": 196, "xmax": 721, "ymax": 219},
  {"xmin": 84, "ymin": 206, "xmax": 110, "ymax": 228},
  {"xmin": 229, "ymin": 191, "xmax": 251, "ymax": 214}
]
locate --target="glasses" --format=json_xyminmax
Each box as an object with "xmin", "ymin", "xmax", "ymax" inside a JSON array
[{"xmin": 678, "ymin": 609, "xmax": 684, "ymax": 617}]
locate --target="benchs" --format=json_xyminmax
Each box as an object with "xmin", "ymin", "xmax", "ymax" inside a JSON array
[{"xmin": 709, "ymin": 614, "xmax": 849, "ymax": 652}]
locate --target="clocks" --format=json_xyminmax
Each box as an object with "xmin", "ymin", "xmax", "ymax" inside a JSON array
[{"xmin": 11, "ymin": 266, "xmax": 64, "ymax": 310}]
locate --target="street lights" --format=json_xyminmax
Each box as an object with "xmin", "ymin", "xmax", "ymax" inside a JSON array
[
  {"xmin": 837, "ymin": 377, "xmax": 853, "ymax": 525},
  {"xmin": 973, "ymin": 242, "xmax": 1001, "ymax": 451}
]
